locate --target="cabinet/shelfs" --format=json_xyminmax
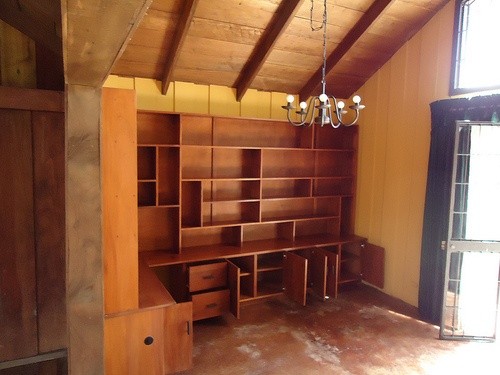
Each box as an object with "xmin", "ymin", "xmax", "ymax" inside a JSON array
[
  {"xmin": 104, "ymin": 300, "xmax": 195, "ymax": 375},
  {"xmin": 136, "ymin": 109, "xmax": 180, "ymax": 265},
  {"xmin": 181, "ymin": 110, "xmax": 368, "ymax": 262},
  {"xmin": 254, "ymin": 249, "xmax": 308, "ymax": 307},
  {"xmin": 101, "ymin": 87, "xmax": 175, "ymax": 318},
  {"xmin": 226, "ymin": 252, "xmax": 257, "ymax": 320},
  {"xmin": 338, "ymin": 238, "xmax": 385, "ymax": 290},
  {"xmin": 316, "ymin": 243, "xmax": 340, "ymax": 300},
  {"xmin": 151, "ymin": 262, "xmax": 186, "ymax": 305},
  {"xmin": 286, "ymin": 247, "xmax": 328, "ymax": 304},
  {"xmin": 186, "ymin": 258, "xmax": 232, "ymax": 324}
]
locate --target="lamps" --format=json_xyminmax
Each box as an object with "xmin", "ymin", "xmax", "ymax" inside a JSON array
[{"xmin": 281, "ymin": 0, "xmax": 367, "ymax": 130}]
